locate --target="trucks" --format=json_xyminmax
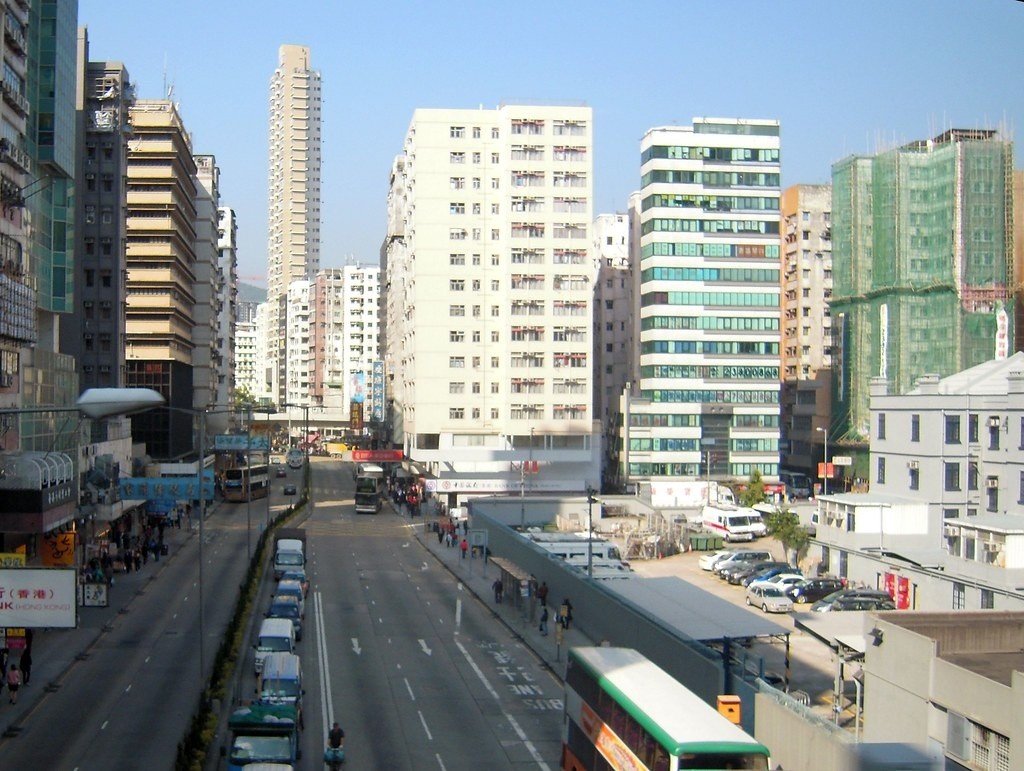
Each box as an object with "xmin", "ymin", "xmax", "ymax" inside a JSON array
[
  {"xmin": 273, "ymin": 528, "xmax": 307, "ymax": 582},
  {"xmin": 219, "ymin": 700, "xmax": 302, "ymax": 771},
  {"xmin": 253, "ymin": 618, "xmax": 296, "ymax": 655},
  {"xmin": 254, "ymin": 653, "xmax": 305, "ymax": 704}
]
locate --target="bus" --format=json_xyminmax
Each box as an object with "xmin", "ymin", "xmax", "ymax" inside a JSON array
[
  {"xmin": 357, "ymin": 463, "xmax": 384, "ymax": 493},
  {"xmin": 558, "ymin": 646, "xmax": 771, "ymax": 771},
  {"xmin": 534, "ymin": 542, "xmax": 636, "ymax": 583},
  {"xmin": 353, "ymin": 476, "xmax": 383, "ymax": 515},
  {"xmin": 223, "ymin": 463, "xmax": 272, "ymax": 502}
]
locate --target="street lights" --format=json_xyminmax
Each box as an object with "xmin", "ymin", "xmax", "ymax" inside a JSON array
[
  {"xmin": 163, "ymin": 405, "xmax": 276, "ymax": 679},
  {"xmin": 817, "ymin": 427, "xmax": 827, "ymax": 493},
  {"xmin": 207, "ymin": 403, "xmax": 273, "ymax": 560},
  {"xmin": 280, "ymin": 404, "xmax": 328, "ymax": 483}
]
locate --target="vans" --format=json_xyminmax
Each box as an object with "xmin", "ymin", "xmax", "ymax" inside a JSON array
[
  {"xmin": 702, "ymin": 503, "xmax": 767, "ymax": 542},
  {"xmin": 786, "ymin": 577, "xmax": 895, "ymax": 611}
]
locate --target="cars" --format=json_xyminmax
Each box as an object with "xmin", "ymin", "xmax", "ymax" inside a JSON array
[
  {"xmin": 276, "ymin": 467, "xmax": 287, "ymax": 477},
  {"xmin": 263, "ymin": 571, "xmax": 307, "ymax": 641},
  {"xmin": 745, "ymin": 581, "xmax": 795, "ymax": 614},
  {"xmin": 271, "ymin": 455, "xmax": 279, "ymax": 464},
  {"xmin": 284, "ymin": 483, "xmax": 296, "ymax": 494},
  {"xmin": 699, "ymin": 550, "xmax": 805, "ymax": 594}
]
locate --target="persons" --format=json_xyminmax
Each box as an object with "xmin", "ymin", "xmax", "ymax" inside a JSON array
[
  {"xmin": 85, "ymin": 551, "xmax": 114, "ymax": 588},
  {"xmin": 25, "ymin": 629, "xmax": 33, "ymax": 649},
  {"xmin": 7, "ymin": 664, "xmax": 20, "ymax": 705},
  {"xmin": 562, "ymin": 599, "xmax": 572, "ymax": 629},
  {"xmin": 170, "ymin": 504, "xmax": 192, "ymax": 529},
  {"xmin": 108, "ymin": 516, "xmax": 168, "ymax": 574},
  {"xmin": 437, "ymin": 516, "xmax": 491, "ymax": 563},
  {"xmin": 392, "ymin": 487, "xmax": 418, "ymax": 514},
  {"xmin": 492, "ymin": 578, "xmax": 503, "ymax": 604},
  {"xmin": 434, "ymin": 501, "xmax": 445, "ymax": 515},
  {"xmin": 19, "ymin": 648, "xmax": 32, "ymax": 686},
  {"xmin": 326, "ymin": 722, "xmax": 345, "ymax": 748},
  {"xmin": 0, "ymin": 647, "xmax": 9, "ymax": 679},
  {"xmin": 539, "ymin": 582, "xmax": 549, "ymax": 606},
  {"xmin": 541, "ymin": 608, "xmax": 549, "ymax": 635},
  {"xmin": 351, "ymin": 375, "xmax": 361, "ymax": 396}
]
